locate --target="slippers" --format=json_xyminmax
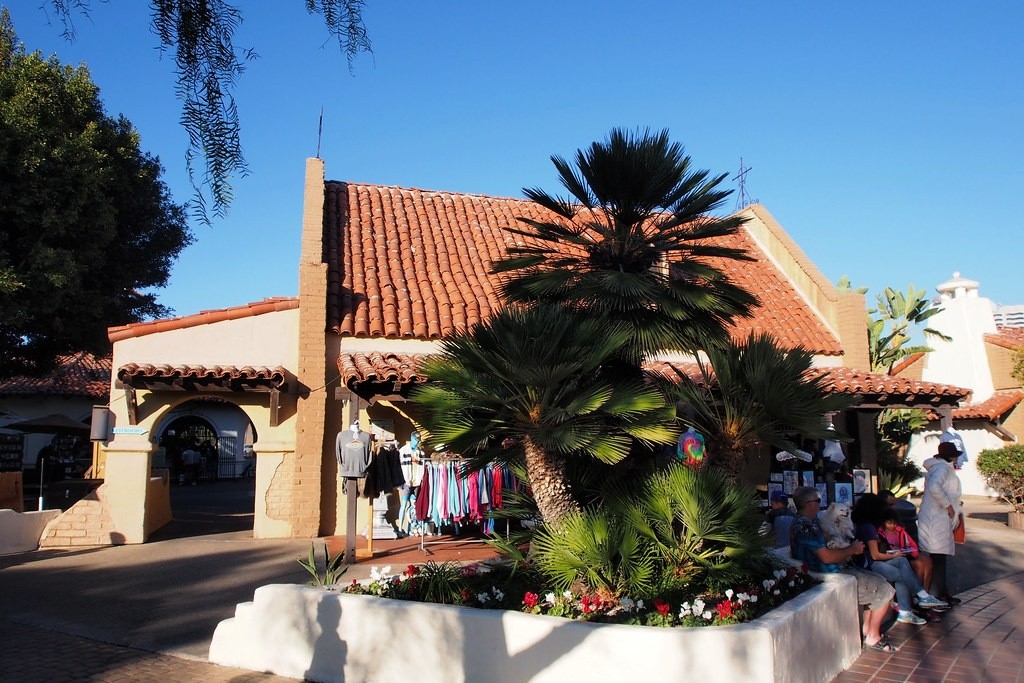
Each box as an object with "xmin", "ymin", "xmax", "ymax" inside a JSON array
[
  {"xmin": 861, "ymin": 639, "xmax": 900, "ymax": 654},
  {"xmin": 861, "ymin": 633, "xmax": 894, "ymax": 644}
]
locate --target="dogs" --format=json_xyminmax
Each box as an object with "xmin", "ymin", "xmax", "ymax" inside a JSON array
[{"xmin": 817, "ymin": 501, "xmax": 855, "ymax": 549}]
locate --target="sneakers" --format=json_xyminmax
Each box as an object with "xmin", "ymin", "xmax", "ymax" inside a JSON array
[
  {"xmin": 896, "ymin": 612, "xmax": 926, "ymax": 625},
  {"xmin": 919, "ymin": 595, "xmax": 948, "ymax": 606}
]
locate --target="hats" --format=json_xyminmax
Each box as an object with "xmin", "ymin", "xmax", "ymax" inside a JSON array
[
  {"xmin": 934, "ymin": 441, "xmax": 963, "ymax": 459},
  {"xmin": 770, "ymin": 491, "xmax": 789, "ymax": 503}
]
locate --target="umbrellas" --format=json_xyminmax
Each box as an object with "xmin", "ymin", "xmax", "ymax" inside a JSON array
[{"xmin": 0, "ymin": 411, "xmax": 91, "ymax": 448}]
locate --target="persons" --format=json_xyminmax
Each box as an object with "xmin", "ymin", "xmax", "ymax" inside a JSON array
[
  {"xmin": 335, "ymin": 425, "xmax": 372, "ymax": 477},
  {"xmin": 788, "ymin": 486, "xmax": 899, "ymax": 653},
  {"xmin": 853, "ymin": 490, "xmax": 946, "ymax": 624},
  {"xmin": 398, "ymin": 431, "xmax": 424, "ymax": 538},
  {"xmin": 36, "ymin": 444, "xmax": 57, "ymax": 485},
  {"xmin": 765, "ymin": 490, "xmax": 794, "ymax": 549},
  {"xmin": 177, "ymin": 441, "xmax": 218, "ymax": 486},
  {"xmin": 916, "ymin": 442, "xmax": 964, "ymax": 610}
]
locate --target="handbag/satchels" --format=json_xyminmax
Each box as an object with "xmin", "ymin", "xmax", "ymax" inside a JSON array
[{"xmin": 953, "ymin": 513, "xmax": 966, "ymax": 544}]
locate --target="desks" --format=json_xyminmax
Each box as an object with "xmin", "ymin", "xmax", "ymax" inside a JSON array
[{"xmin": 752, "ymin": 484, "xmax": 767, "ymax": 507}]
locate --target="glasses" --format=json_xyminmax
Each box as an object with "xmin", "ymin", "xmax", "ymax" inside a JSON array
[{"xmin": 805, "ymin": 497, "xmax": 821, "ymax": 503}]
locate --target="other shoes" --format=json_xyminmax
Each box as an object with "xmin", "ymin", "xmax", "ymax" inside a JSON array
[
  {"xmin": 932, "ymin": 597, "xmax": 947, "ymax": 612},
  {"xmin": 927, "ymin": 610, "xmax": 941, "ymax": 622},
  {"xmin": 936, "ymin": 593, "xmax": 961, "ymax": 606}
]
